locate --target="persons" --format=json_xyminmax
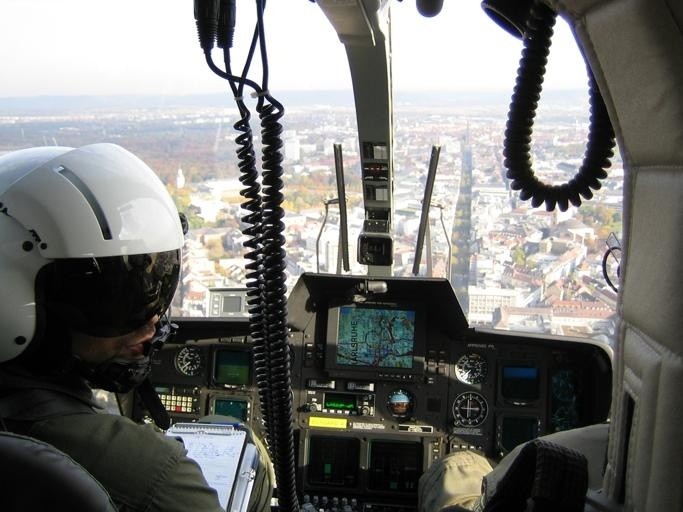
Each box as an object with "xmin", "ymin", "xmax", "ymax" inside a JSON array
[
  {"xmin": 415, "ymin": 420, "xmax": 610, "ymax": 510},
  {"xmin": 0, "ymin": 142, "xmax": 222, "ymax": 512}
]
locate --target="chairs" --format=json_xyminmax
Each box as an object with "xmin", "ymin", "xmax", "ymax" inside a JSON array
[{"xmin": 0, "ymin": 430, "xmax": 120, "ymax": 512}]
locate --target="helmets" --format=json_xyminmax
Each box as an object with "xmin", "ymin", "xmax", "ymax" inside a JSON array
[{"xmin": 0, "ymin": 143, "xmax": 188, "ymax": 362}]
